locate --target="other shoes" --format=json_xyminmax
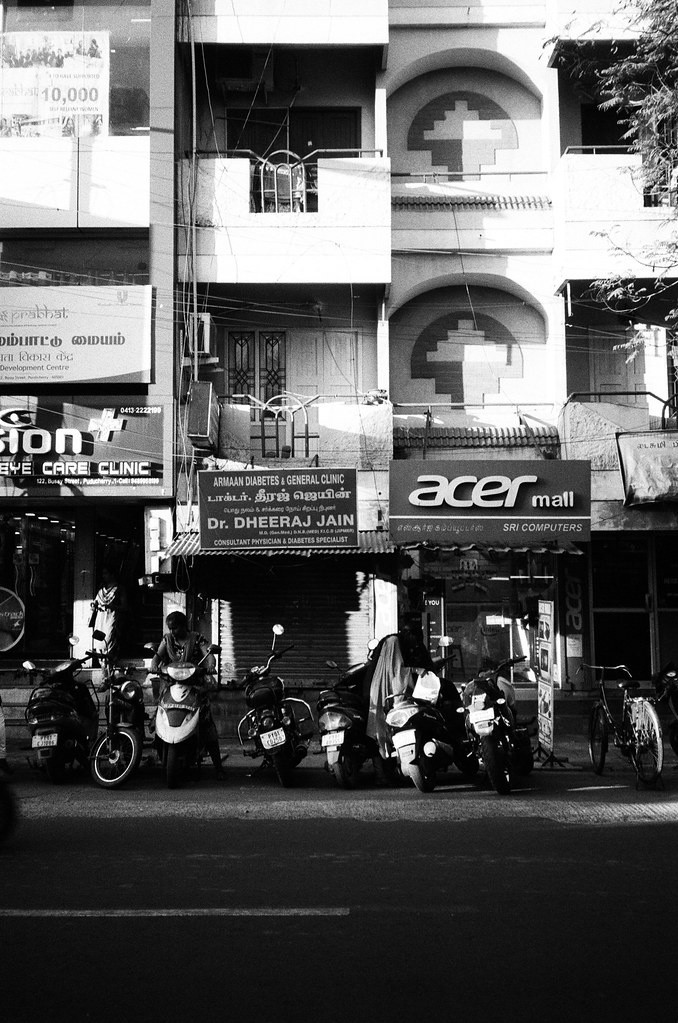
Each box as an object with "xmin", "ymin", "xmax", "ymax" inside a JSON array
[
  {"xmin": 377, "ymin": 779, "xmax": 387, "ymax": 789},
  {"xmin": 386, "ymin": 776, "xmax": 404, "ymax": 784},
  {"xmin": 215, "ymin": 764, "xmax": 226, "ymax": 781}
]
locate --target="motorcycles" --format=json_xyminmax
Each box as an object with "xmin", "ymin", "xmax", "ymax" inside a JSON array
[
  {"xmin": 228, "ymin": 623, "xmax": 315, "ymax": 788},
  {"xmin": 379, "ymin": 634, "xmax": 480, "ymax": 794},
  {"xmin": 143, "ymin": 641, "xmax": 222, "ymax": 790},
  {"xmin": 85, "ymin": 630, "xmax": 151, "ymax": 790},
  {"xmin": 15, "ymin": 634, "xmax": 101, "ymax": 784},
  {"xmin": 457, "ymin": 656, "xmax": 538, "ymax": 795},
  {"xmin": 315, "ymin": 639, "xmax": 379, "ymax": 790},
  {"xmin": 653, "ymin": 658, "xmax": 678, "ymax": 763}
]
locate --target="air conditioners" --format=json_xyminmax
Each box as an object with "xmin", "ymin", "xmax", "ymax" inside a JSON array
[
  {"xmin": 213, "ymin": 45, "xmax": 275, "ymax": 92},
  {"xmin": 187, "ymin": 312, "xmax": 217, "ymax": 357}
]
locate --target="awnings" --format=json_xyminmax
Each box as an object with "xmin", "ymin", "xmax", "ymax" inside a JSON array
[
  {"xmin": 165, "ymin": 531, "xmax": 395, "ymax": 555},
  {"xmin": 400, "ymin": 536, "xmax": 585, "ymax": 555}
]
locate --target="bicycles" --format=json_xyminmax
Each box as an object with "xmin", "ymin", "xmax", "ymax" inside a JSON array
[{"xmin": 576, "ymin": 663, "xmax": 667, "ymax": 792}]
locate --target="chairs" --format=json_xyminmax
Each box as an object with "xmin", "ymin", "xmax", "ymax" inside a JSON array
[{"xmin": 259, "ymin": 164, "xmax": 304, "ymax": 211}]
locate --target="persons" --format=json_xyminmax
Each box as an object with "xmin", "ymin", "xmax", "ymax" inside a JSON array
[
  {"xmin": 149, "ymin": 612, "xmax": 229, "ymax": 782},
  {"xmin": 90, "ymin": 566, "xmax": 122, "ymax": 667}
]
